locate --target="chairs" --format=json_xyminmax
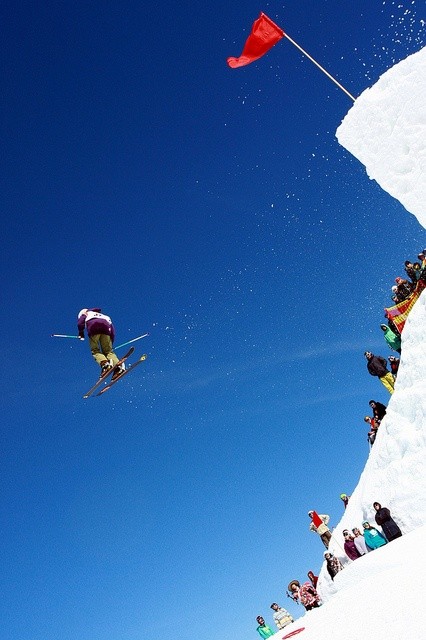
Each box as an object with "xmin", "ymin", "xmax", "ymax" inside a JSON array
[
  {"xmin": 110, "ymin": 366, "xmax": 122, "ymax": 381},
  {"xmin": 100, "ymin": 361, "xmax": 109, "ymax": 376}
]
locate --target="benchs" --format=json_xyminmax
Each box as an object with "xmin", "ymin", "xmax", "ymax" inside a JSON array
[
  {"xmin": 226, "ymin": 10, "xmax": 285, "ymax": 69},
  {"xmin": 383, "ymin": 279, "xmax": 426, "ymax": 335},
  {"xmin": 312, "ymin": 511, "xmax": 323, "ymax": 528}
]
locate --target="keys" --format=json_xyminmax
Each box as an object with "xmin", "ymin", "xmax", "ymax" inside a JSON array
[{"xmin": 282, "ymin": 628, "xmax": 304, "ymax": 639}]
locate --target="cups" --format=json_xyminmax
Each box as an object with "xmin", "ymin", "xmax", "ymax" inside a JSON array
[
  {"xmin": 364, "ymin": 353, "xmax": 369, "ymax": 358},
  {"xmin": 391, "ymin": 297, "xmax": 395, "ymax": 300},
  {"xmin": 270, "ymin": 603, "xmax": 275, "ymax": 607},
  {"xmin": 369, "ymin": 403, "xmax": 374, "ymax": 407},
  {"xmin": 373, "ymin": 504, "xmax": 377, "ymax": 507},
  {"xmin": 256, "ymin": 616, "xmax": 260, "ymax": 619},
  {"xmin": 342, "ymin": 532, "xmax": 348, "ymax": 536}
]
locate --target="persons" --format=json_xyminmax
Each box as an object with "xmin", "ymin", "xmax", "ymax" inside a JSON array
[
  {"xmin": 364, "ymin": 416, "xmax": 380, "ymax": 445},
  {"xmin": 369, "ymin": 399, "xmax": 386, "ymax": 424},
  {"xmin": 270, "ymin": 603, "xmax": 294, "ymax": 630},
  {"xmin": 322, "ymin": 550, "xmax": 344, "ymax": 581},
  {"xmin": 385, "ymin": 314, "xmax": 401, "ymax": 337},
  {"xmin": 364, "ymin": 351, "xmax": 395, "ymax": 395},
  {"xmin": 404, "ymin": 261, "xmax": 421, "ymax": 287},
  {"xmin": 373, "ymin": 501, "xmax": 402, "ymax": 542},
  {"xmin": 352, "ymin": 528, "xmax": 372, "ymax": 556},
  {"xmin": 392, "ymin": 286, "xmax": 406, "ymax": 302},
  {"xmin": 339, "ymin": 493, "xmax": 351, "ymax": 509},
  {"xmin": 342, "ymin": 529, "xmax": 362, "ymax": 561},
  {"xmin": 307, "ymin": 510, "xmax": 332, "ymax": 550},
  {"xmin": 362, "ymin": 520, "xmax": 387, "ymax": 550},
  {"xmin": 307, "ymin": 570, "xmax": 318, "ymax": 589},
  {"xmin": 391, "ymin": 296, "xmax": 401, "ymax": 304},
  {"xmin": 380, "ymin": 323, "xmax": 401, "ymax": 355},
  {"xmin": 395, "ymin": 277, "xmax": 413, "ymax": 297},
  {"xmin": 77, "ymin": 307, "xmax": 125, "ymax": 381},
  {"xmin": 388, "ymin": 355, "xmax": 400, "ymax": 376},
  {"xmin": 256, "ymin": 615, "xmax": 273, "ymax": 640},
  {"xmin": 287, "ymin": 579, "xmax": 320, "ymax": 611},
  {"xmin": 418, "ymin": 253, "xmax": 426, "ymax": 269}
]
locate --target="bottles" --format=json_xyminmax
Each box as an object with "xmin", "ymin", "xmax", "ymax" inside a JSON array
[
  {"xmin": 307, "ymin": 511, "xmax": 313, "ymax": 515},
  {"xmin": 322, "ymin": 550, "xmax": 334, "ymax": 559},
  {"xmin": 287, "ymin": 580, "xmax": 299, "ymax": 592},
  {"xmin": 395, "ymin": 277, "xmax": 401, "ymax": 283},
  {"xmin": 271, "ymin": 602, "xmax": 278, "ymax": 610},
  {"xmin": 256, "ymin": 616, "xmax": 263, "ymax": 623},
  {"xmin": 404, "ymin": 261, "xmax": 410, "ymax": 266},
  {"xmin": 362, "ymin": 520, "xmax": 368, "ymax": 525},
  {"xmin": 417, "ymin": 253, "xmax": 425, "ymax": 261},
  {"xmin": 391, "ymin": 286, "xmax": 397, "ymax": 292},
  {"xmin": 339, "ymin": 494, "xmax": 346, "ymax": 500},
  {"xmin": 390, "ymin": 295, "xmax": 395, "ymax": 300}
]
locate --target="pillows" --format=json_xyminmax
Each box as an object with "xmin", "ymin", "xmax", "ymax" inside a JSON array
[
  {"xmin": 364, "ymin": 352, "xmax": 370, "ymax": 356},
  {"xmin": 364, "ymin": 416, "xmax": 370, "ymax": 422}
]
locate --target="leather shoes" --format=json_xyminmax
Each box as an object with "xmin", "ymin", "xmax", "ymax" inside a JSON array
[{"xmin": 78, "ymin": 331, "xmax": 84, "ymax": 339}]
